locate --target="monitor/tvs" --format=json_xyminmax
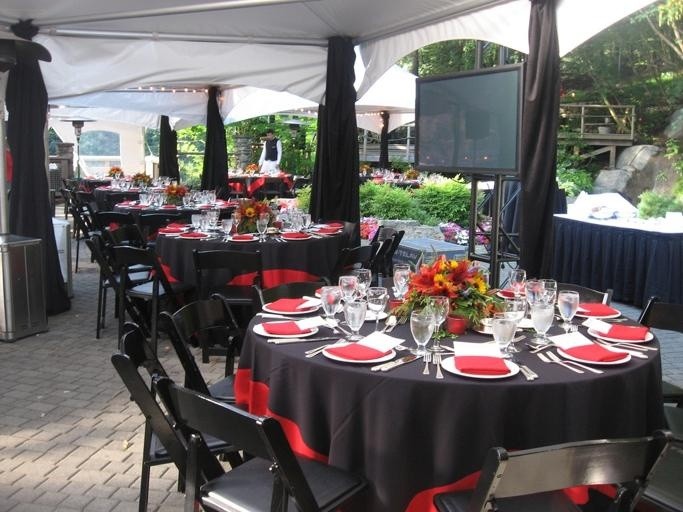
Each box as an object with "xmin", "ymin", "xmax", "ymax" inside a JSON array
[{"xmin": 414, "ymin": 63, "xmax": 525, "ymax": 176}]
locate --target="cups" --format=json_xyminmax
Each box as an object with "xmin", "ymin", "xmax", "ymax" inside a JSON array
[{"xmin": 480, "ymin": 318, "xmax": 492, "ymax": 332}]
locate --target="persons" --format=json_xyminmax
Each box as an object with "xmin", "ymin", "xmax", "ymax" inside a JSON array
[{"xmin": 256, "ymin": 129, "xmax": 283, "ymax": 176}]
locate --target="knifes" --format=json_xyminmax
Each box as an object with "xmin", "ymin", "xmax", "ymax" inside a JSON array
[
  {"xmin": 371, "ymin": 352, "xmax": 432, "ymax": 372},
  {"xmin": 591, "ymin": 340, "xmax": 647, "ymax": 359}
]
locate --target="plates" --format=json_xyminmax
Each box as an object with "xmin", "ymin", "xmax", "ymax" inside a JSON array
[
  {"xmin": 252, "ymin": 321, "xmax": 319, "ymax": 339},
  {"xmin": 343, "ymin": 299, "xmax": 366, "ymax": 341},
  {"xmin": 280, "ymin": 235, "xmax": 312, "ymax": 241},
  {"xmin": 440, "ymin": 354, "xmax": 519, "ymax": 379},
  {"xmin": 227, "ymin": 236, "xmax": 259, "ymax": 242},
  {"xmin": 178, "ymin": 235, "xmax": 210, "ymax": 239},
  {"xmin": 158, "ymin": 230, "xmax": 188, "ymax": 235},
  {"xmin": 556, "ymin": 344, "xmax": 631, "ymax": 365},
  {"xmin": 586, "ymin": 324, "xmax": 653, "ymax": 344},
  {"xmin": 312, "ymin": 231, "xmax": 340, "ymax": 235},
  {"xmin": 472, "ymin": 323, "xmax": 492, "ymax": 335},
  {"xmin": 261, "ymin": 301, "xmax": 319, "ymax": 315},
  {"xmin": 574, "ymin": 307, "xmax": 621, "ymax": 320},
  {"xmin": 322, "ymin": 342, "xmax": 396, "ymax": 363}
]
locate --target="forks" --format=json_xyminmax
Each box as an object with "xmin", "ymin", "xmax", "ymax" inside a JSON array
[
  {"xmin": 387, "ymin": 315, "xmax": 407, "ymax": 334},
  {"xmin": 536, "ymin": 352, "xmax": 584, "ymax": 374},
  {"xmin": 545, "ymin": 351, "xmax": 603, "ymax": 374},
  {"xmin": 596, "ymin": 338, "xmax": 657, "ymax": 351}
]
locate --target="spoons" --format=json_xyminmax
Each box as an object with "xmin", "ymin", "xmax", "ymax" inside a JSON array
[{"xmin": 381, "ymin": 316, "xmax": 395, "ymax": 333}]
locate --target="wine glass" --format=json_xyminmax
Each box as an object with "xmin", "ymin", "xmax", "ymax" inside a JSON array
[
  {"xmin": 394, "ymin": 270, "xmax": 409, "ymax": 300},
  {"xmin": 320, "ymin": 286, "xmax": 341, "ymax": 327},
  {"xmin": 221, "ymin": 219, "xmax": 232, "ymax": 242},
  {"xmin": 427, "ymin": 296, "xmax": 449, "ymax": 352},
  {"xmin": 508, "ymin": 268, "xmax": 526, "ymax": 297},
  {"xmin": 110, "ymin": 173, "xmax": 131, "ymax": 191},
  {"xmin": 530, "ymin": 302, "xmax": 554, "ymax": 345},
  {"xmin": 392, "ymin": 263, "xmax": 410, "ymax": 291},
  {"xmin": 557, "ymin": 289, "xmax": 579, "ymax": 333},
  {"xmin": 410, "ymin": 309, "xmax": 434, "ymax": 356},
  {"xmin": 276, "ymin": 208, "xmax": 312, "ymax": 233},
  {"xmin": 191, "ymin": 208, "xmax": 220, "ymax": 232},
  {"xmin": 231, "ymin": 212, "xmax": 241, "ymax": 236},
  {"xmin": 182, "ymin": 189, "xmax": 216, "ymax": 207},
  {"xmin": 260, "ymin": 212, "xmax": 268, "ymax": 229},
  {"xmin": 366, "ymin": 286, "xmax": 387, "ymax": 332},
  {"xmin": 350, "ymin": 268, "xmax": 372, "ymax": 303},
  {"xmin": 256, "ymin": 219, "xmax": 266, "ymax": 243},
  {"xmin": 491, "ymin": 312, "xmax": 515, "ymax": 359},
  {"xmin": 338, "ymin": 276, "xmax": 358, "ymax": 325},
  {"xmin": 503, "ymin": 297, "xmax": 526, "ymax": 352},
  {"xmin": 152, "ymin": 176, "xmax": 178, "ymax": 187},
  {"xmin": 524, "ymin": 277, "xmax": 557, "ymax": 306},
  {"xmin": 137, "ymin": 191, "xmax": 164, "ymax": 207}
]
